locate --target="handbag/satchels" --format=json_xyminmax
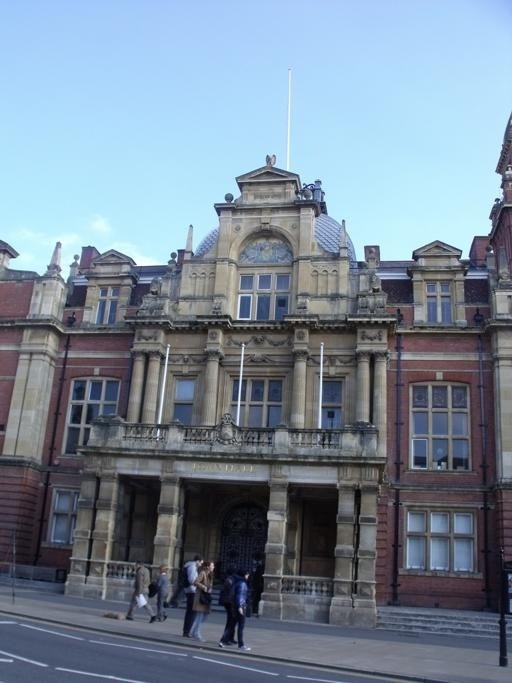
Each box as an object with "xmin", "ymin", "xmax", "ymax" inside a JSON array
[
  {"xmin": 199, "ymin": 591, "xmax": 212, "ymax": 604},
  {"xmin": 149, "ymin": 582, "xmax": 159, "ymax": 598}
]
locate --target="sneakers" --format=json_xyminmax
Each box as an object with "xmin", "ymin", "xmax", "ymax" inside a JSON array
[
  {"xmin": 239, "ymin": 646, "xmax": 250, "ymax": 650},
  {"xmin": 150, "ymin": 616, "xmax": 156, "ymax": 623}
]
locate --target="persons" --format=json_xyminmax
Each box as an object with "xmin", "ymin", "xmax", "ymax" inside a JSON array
[
  {"xmin": 154, "ymin": 563, "xmax": 170, "ymax": 623},
  {"xmin": 178, "ymin": 554, "xmax": 264, "ymax": 651},
  {"xmin": 126, "ymin": 561, "xmax": 156, "ymax": 623}
]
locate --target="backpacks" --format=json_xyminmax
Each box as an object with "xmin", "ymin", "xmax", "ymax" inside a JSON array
[
  {"xmin": 223, "ymin": 576, "xmax": 235, "ymax": 608},
  {"xmin": 178, "ymin": 566, "xmax": 191, "ymax": 588}
]
